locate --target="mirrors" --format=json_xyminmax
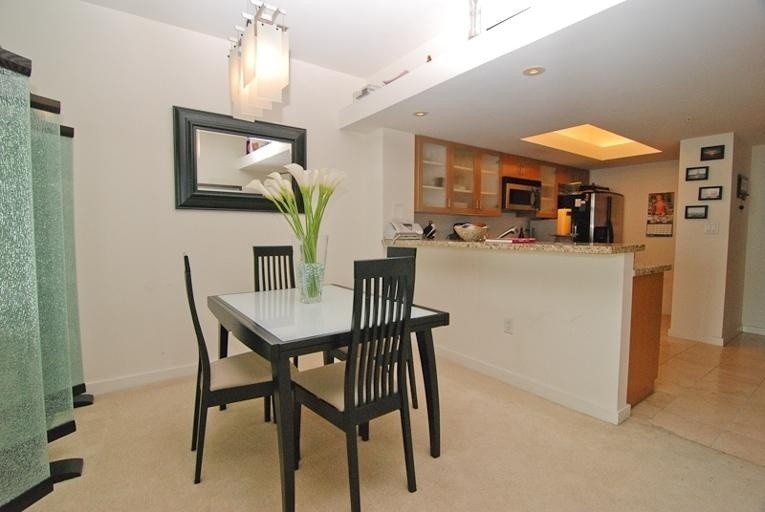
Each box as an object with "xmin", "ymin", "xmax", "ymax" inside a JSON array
[{"xmin": 173, "ymin": 106, "xmax": 307, "ymax": 213}]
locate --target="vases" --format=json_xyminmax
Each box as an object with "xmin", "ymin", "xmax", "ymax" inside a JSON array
[{"xmin": 296, "ymin": 234, "xmax": 329, "ymax": 304}]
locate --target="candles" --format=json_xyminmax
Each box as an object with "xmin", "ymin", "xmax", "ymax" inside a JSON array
[{"xmin": 556, "ymin": 206, "xmax": 572, "ymax": 236}]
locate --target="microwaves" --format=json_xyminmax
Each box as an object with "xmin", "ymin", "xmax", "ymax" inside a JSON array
[{"xmin": 503, "ymin": 182, "xmax": 541, "ymax": 212}]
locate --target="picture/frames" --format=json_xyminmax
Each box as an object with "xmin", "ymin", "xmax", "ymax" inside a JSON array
[
  {"xmin": 700, "ymin": 145, "xmax": 724, "ymax": 160},
  {"xmin": 685, "ymin": 205, "xmax": 707, "ymax": 219},
  {"xmin": 685, "ymin": 166, "xmax": 709, "ymax": 181},
  {"xmin": 698, "ymin": 186, "xmax": 722, "ymax": 199},
  {"xmin": 737, "ymin": 173, "xmax": 750, "ymax": 201}
]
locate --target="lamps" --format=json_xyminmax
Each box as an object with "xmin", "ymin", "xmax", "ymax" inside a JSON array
[{"xmin": 225, "ymin": 0, "xmax": 290, "ymax": 123}]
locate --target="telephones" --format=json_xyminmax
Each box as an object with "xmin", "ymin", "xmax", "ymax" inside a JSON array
[{"xmin": 388, "ymin": 222, "xmax": 424, "ymax": 239}]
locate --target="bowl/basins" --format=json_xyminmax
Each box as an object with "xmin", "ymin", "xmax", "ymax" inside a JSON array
[{"xmin": 454, "ymin": 225, "xmax": 486, "ymax": 242}]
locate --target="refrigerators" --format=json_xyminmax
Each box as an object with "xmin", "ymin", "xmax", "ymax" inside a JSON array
[{"xmin": 556, "ymin": 191, "xmax": 623, "ymax": 244}]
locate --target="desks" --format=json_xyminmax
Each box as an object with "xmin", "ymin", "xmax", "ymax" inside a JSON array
[{"xmin": 207, "ymin": 283, "xmax": 449, "ymax": 512}]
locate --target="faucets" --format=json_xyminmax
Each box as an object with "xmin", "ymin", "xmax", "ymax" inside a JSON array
[{"xmin": 498, "ymin": 227, "xmax": 519, "ymax": 238}]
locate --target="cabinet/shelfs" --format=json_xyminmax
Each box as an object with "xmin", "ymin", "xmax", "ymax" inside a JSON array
[{"xmin": 414, "ymin": 133, "xmax": 590, "ymax": 220}]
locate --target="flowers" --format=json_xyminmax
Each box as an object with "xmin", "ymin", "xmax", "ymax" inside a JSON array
[{"xmin": 242, "ymin": 163, "xmax": 346, "ymax": 297}]
